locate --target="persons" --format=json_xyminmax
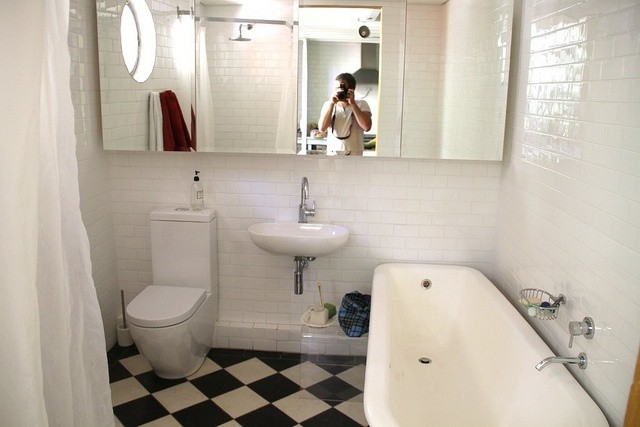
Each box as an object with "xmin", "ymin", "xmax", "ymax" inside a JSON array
[{"xmin": 318, "ymin": 72, "xmax": 373, "ymax": 157}]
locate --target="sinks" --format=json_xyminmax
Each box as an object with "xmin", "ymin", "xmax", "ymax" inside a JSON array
[{"xmin": 249, "ymin": 221, "xmax": 347, "ymax": 256}]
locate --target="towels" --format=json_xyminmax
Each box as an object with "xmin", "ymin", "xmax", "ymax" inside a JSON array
[
  {"xmin": 148, "ymin": 91, "xmax": 163, "ymax": 152},
  {"xmin": 159, "ymin": 89, "xmax": 192, "ymax": 151}
]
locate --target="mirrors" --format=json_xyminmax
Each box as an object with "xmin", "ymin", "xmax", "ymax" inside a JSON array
[{"xmin": 94, "ymin": 1, "xmax": 515, "ymax": 163}]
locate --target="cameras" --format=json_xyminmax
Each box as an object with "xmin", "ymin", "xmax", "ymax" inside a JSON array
[{"xmin": 336, "ymin": 82, "xmax": 348, "ymax": 100}]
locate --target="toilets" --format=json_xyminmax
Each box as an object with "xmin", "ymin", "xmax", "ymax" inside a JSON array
[{"xmin": 126, "ymin": 208, "xmax": 220, "ymax": 381}]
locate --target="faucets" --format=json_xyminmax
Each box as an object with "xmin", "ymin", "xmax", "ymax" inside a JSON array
[
  {"xmin": 535, "ymin": 352, "xmax": 587, "ymax": 372},
  {"xmin": 299, "ymin": 177, "xmax": 315, "ymax": 223}
]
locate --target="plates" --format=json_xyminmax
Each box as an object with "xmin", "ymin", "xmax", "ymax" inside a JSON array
[{"xmin": 300, "ymin": 307, "xmax": 337, "ymax": 327}]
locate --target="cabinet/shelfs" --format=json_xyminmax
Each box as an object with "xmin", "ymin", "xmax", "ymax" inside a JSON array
[{"xmin": 297, "ymin": 134, "xmax": 327, "ymax": 155}]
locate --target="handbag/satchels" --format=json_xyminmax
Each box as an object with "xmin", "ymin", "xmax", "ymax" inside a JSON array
[{"xmin": 338, "ymin": 291, "xmax": 370, "ymax": 336}]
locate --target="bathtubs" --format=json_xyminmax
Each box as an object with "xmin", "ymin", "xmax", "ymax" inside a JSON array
[{"xmin": 364, "ymin": 262, "xmax": 609, "ymax": 427}]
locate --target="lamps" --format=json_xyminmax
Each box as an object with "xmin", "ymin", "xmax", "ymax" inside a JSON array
[{"xmin": 358, "ymin": 25, "xmax": 370, "ymax": 38}]
